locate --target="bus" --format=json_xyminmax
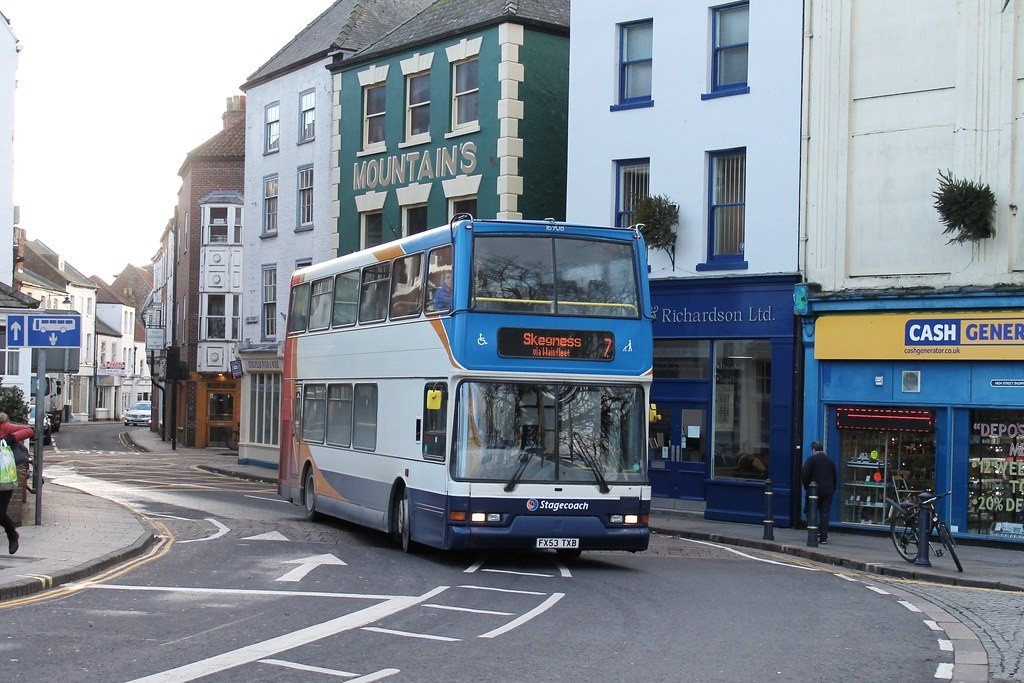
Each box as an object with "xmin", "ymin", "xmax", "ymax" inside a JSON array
[{"xmin": 277, "ymin": 212, "xmax": 653, "ymax": 565}]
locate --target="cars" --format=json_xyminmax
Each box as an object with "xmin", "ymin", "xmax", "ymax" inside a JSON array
[
  {"xmin": 123, "ymin": 400, "xmax": 152, "ymax": 427},
  {"xmin": 27, "ymin": 404, "xmax": 53, "ymax": 445}
]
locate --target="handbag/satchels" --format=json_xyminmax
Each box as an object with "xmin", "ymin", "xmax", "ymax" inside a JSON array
[
  {"xmin": 8, "ymin": 441, "xmax": 28, "ymax": 464},
  {"xmin": 0, "ymin": 439, "xmax": 18, "ymax": 491}
]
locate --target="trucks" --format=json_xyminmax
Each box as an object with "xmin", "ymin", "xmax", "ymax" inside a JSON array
[{"xmin": 31, "ymin": 371, "xmax": 62, "ymax": 433}]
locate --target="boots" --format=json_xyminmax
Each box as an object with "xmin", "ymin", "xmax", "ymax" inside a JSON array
[{"xmin": 4, "ymin": 527, "xmax": 19, "ymax": 554}]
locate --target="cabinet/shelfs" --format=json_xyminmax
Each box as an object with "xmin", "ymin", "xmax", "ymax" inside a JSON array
[{"xmin": 844, "ymin": 431, "xmax": 936, "ymax": 526}]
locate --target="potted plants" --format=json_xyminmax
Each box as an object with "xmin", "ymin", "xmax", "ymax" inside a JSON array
[
  {"xmin": 932, "ymin": 169, "xmax": 995, "ymax": 246},
  {"xmin": 628, "ymin": 193, "xmax": 679, "ymax": 250}
]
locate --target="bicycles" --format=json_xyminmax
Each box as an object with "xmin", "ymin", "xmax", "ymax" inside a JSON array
[{"xmin": 890, "ymin": 490, "xmax": 965, "ymax": 574}]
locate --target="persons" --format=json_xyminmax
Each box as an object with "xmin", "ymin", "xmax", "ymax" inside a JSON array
[
  {"xmin": 802, "ymin": 441, "xmax": 837, "ymax": 543},
  {"xmin": 0, "ymin": 412, "xmax": 34, "ymax": 554},
  {"xmin": 433, "ymin": 272, "xmax": 452, "ymax": 311}
]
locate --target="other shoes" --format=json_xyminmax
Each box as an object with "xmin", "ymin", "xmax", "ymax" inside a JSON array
[
  {"xmin": 820, "ymin": 538, "xmax": 827, "ymax": 544},
  {"xmin": 817, "ymin": 533, "xmax": 820, "ymax": 537}
]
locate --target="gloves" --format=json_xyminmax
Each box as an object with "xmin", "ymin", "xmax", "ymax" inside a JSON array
[{"xmin": 2, "ymin": 434, "xmax": 15, "ymax": 446}]
